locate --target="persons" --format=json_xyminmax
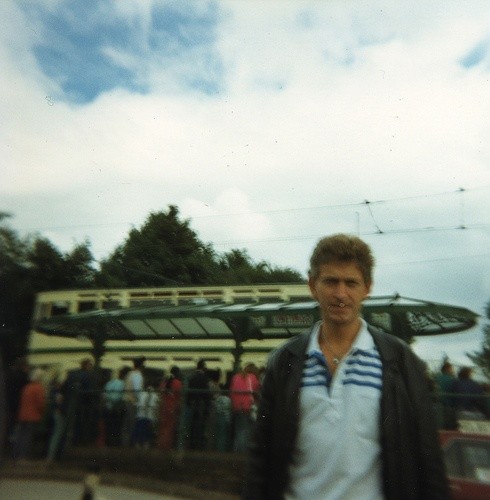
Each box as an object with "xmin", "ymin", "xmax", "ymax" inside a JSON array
[
  {"xmin": 71, "ymin": 367, "xmax": 117, "ymax": 500},
  {"xmin": 14, "ymin": 350, "xmax": 476, "ymax": 473},
  {"xmin": 241, "ymin": 233, "xmax": 455, "ymax": 500}
]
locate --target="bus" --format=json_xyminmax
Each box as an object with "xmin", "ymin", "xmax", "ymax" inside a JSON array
[{"xmin": 26, "ymin": 284, "xmax": 322, "ymax": 394}]
